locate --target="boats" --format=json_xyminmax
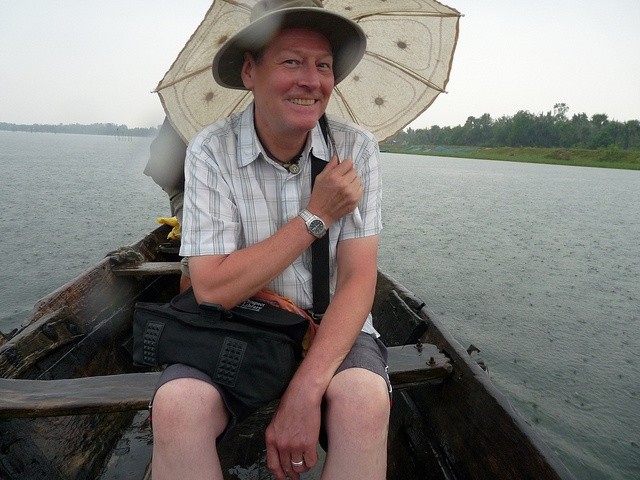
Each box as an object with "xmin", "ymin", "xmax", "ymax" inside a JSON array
[{"xmin": 1, "ymin": 219, "xmax": 574, "ymax": 476}]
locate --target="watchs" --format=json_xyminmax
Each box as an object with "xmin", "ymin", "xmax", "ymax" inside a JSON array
[{"xmin": 297, "ymin": 209, "xmax": 327, "ymax": 240}]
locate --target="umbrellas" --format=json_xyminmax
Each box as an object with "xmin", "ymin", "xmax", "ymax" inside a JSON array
[{"xmin": 147, "ymin": 0, "xmax": 466, "ymax": 227}]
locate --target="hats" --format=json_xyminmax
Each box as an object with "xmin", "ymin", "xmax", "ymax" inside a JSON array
[{"xmin": 211, "ymin": 1, "xmax": 367, "ymax": 91}]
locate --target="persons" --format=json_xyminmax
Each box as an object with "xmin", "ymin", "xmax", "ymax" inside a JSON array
[
  {"xmin": 142, "ymin": 115, "xmax": 191, "ymax": 294},
  {"xmin": 148, "ymin": 0, "xmax": 392, "ymax": 478}
]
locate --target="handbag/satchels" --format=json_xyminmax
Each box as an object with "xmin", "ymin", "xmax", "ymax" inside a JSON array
[{"xmin": 131, "ymin": 115, "xmax": 332, "ymax": 422}]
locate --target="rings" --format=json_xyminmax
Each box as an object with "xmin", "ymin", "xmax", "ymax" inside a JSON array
[{"xmin": 290, "ymin": 459, "xmax": 304, "ymax": 467}]
